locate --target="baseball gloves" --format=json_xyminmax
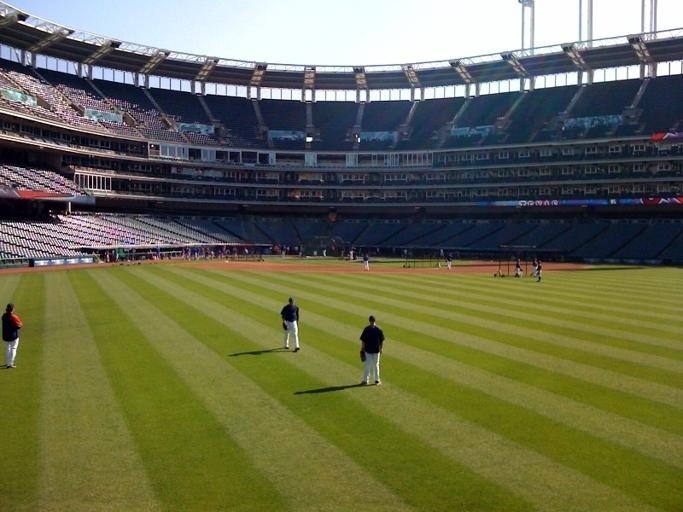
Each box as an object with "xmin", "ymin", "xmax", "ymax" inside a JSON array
[
  {"xmin": 360, "ymin": 351, "xmax": 367, "ymax": 362},
  {"xmin": 282, "ymin": 320, "xmax": 289, "ymax": 331}
]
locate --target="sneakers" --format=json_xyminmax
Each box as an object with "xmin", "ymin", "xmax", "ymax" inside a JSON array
[
  {"xmin": 361, "ymin": 380, "xmax": 380, "ymax": 385},
  {"xmin": 285, "ymin": 346, "xmax": 300, "ymax": 352},
  {"xmin": 5, "ymin": 364, "xmax": 17, "ymax": 368}
]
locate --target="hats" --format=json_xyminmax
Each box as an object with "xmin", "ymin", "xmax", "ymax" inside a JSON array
[
  {"xmin": 7, "ymin": 303, "xmax": 14, "ymax": 308},
  {"xmin": 369, "ymin": 316, "xmax": 375, "ymax": 321},
  {"xmin": 289, "ymin": 298, "xmax": 293, "ymax": 302}
]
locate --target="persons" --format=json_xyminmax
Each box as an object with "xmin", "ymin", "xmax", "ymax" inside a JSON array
[
  {"xmin": 359, "ymin": 314, "xmax": 386, "ymax": 386},
  {"xmin": 279, "ymin": 296, "xmax": 302, "ymax": 354},
  {"xmin": 0, "ymin": 302, "xmax": 22, "ymax": 370}
]
